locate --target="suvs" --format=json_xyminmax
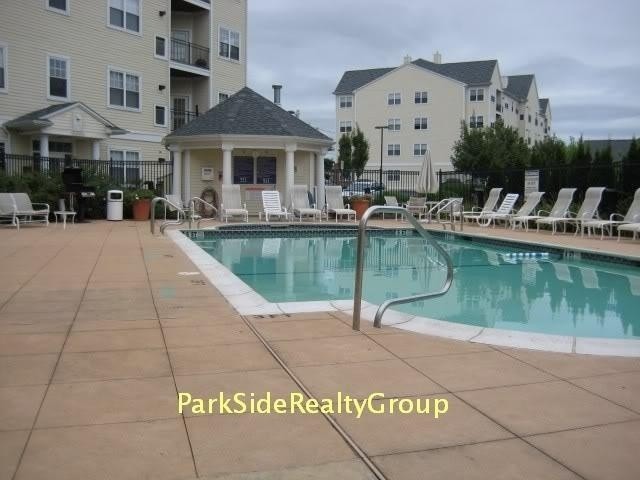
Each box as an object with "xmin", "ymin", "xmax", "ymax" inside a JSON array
[{"xmin": 342, "ymin": 178, "xmax": 386, "ymax": 203}]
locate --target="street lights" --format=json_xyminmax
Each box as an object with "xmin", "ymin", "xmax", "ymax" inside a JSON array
[
  {"xmin": 373, "ymin": 240, "xmax": 387, "ymax": 278},
  {"xmin": 374, "ymin": 124, "xmax": 390, "ymax": 215}
]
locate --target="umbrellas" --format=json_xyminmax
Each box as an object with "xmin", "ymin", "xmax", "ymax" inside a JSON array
[{"xmin": 415, "ymin": 146, "xmax": 440, "ymax": 206}]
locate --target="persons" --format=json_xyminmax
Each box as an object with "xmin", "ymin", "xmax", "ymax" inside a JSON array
[{"xmin": 360, "ymin": 187, "xmax": 372, "ymax": 200}]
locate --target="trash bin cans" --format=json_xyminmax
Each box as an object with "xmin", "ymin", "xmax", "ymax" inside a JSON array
[{"xmin": 107, "ymin": 189, "xmax": 124, "ymax": 221}]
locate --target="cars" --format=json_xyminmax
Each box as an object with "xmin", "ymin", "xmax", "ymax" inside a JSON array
[{"xmin": 325, "ymin": 174, "xmax": 340, "ymax": 185}]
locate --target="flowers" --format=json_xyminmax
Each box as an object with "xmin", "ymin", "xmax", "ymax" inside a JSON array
[{"xmin": 349, "ymin": 192, "xmax": 373, "ymax": 200}]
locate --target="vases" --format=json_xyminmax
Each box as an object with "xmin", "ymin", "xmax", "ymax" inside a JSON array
[{"xmin": 351, "ymin": 200, "xmax": 370, "ymax": 219}]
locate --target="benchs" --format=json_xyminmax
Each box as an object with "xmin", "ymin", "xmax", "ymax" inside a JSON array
[{"xmin": 0, "ymin": 192, "xmax": 50, "ymax": 231}]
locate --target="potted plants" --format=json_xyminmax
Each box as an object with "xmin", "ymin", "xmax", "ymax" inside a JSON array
[{"xmin": 123, "ymin": 188, "xmax": 155, "ymax": 220}]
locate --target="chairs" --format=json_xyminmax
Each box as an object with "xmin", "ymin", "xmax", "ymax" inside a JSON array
[
  {"xmin": 393, "ymin": 228, "xmax": 640, "ymax": 297},
  {"xmin": 220, "ymin": 184, "xmax": 249, "ymax": 223},
  {"xmin": 220, "ymin": 237, "xmax": 359, "ymax": 264},
  {"xmin": 382, "ymin": 186, "xmax": 640, "ymax": 244},
  {"xmin": 290, "ymin": 184, "xmax": 322, "ymax": 222},
  {"xmin": 164, "ymin": 194, "xmax": 194, "ymax": 223},
  {"xmin": 325, "ymin": 185, "xmax": 357, "ymax": 223},
  {"xmin": 258, "ymin": 190, "xmax": 291, "ymax": 222}
]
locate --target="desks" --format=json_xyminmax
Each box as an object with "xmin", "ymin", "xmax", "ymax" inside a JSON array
[{"xmin": 53, "ymin": 210, "xmax": 77, "ymax": 229}]
